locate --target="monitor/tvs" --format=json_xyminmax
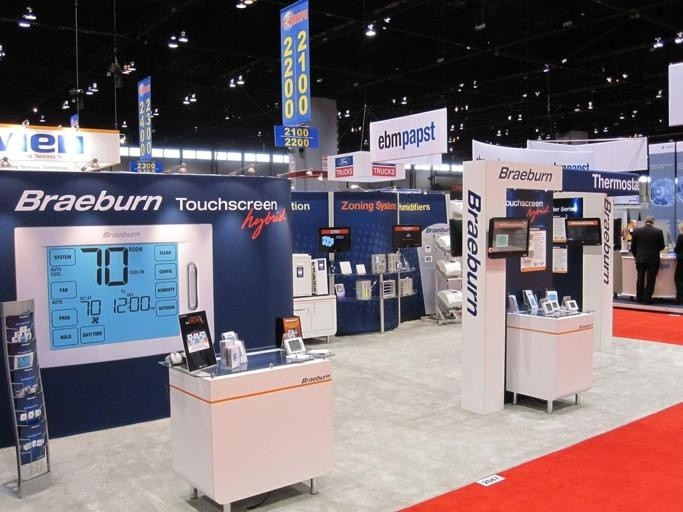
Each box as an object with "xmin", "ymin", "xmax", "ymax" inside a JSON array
[
  {"xmin": 392, "ymin": 224, "xmax": 422, "ymax": 247},
  {"xmin": 488, "ymin": 216, "xmax": 530, "ymax": 258},
  {"xmin": 565, "ymin": 218, "xmax": 603, "ymax": 246},
  {"xmin": 449, "ymin": 218, "xmax": 462, "ymax": 257},
  {"xmin": 318, "ymin": 226, "xmax": 351, "ymax": 252}
]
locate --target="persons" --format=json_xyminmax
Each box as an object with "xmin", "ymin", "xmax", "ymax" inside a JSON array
[
  {"xmin": 631, "ymin": 216, "xmax": 665, "ymax": 305},
  {"xmin": 672, "ymin": 223, "xmax": 683, "ymax": 305}
]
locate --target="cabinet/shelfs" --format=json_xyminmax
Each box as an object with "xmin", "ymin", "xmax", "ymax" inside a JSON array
[
  {"xmin": 293, "ymin": 294, "xmax": 337, "ymax": 344},
  {"xmin": 434, "ymin": 233, "xmax": 462, "ymax": 325},
  {"xmin": 331, "ymin": 264, "xmax": 416, "ymax": 334}
]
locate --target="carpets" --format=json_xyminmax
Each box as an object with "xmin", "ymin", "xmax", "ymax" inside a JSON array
[
  {"xmin": 611, "ymin": 307, "xmax": 683, "ymax": 347},
  {"xmin": 392, "ymin": 403, "xmax": 682, "ymax": 510}
]
